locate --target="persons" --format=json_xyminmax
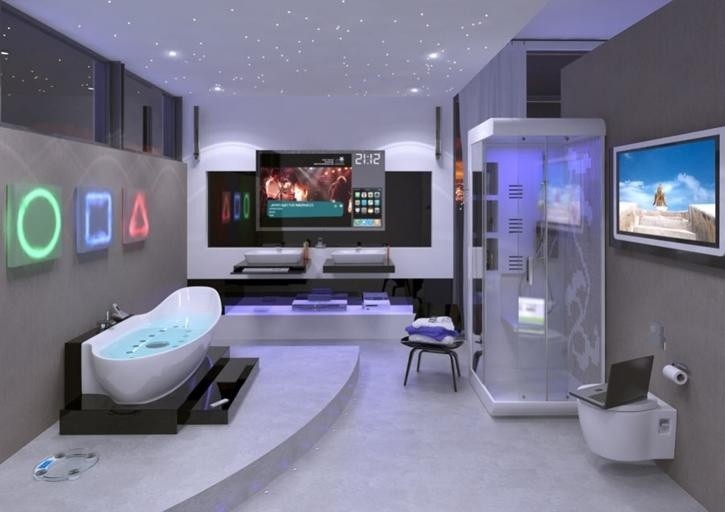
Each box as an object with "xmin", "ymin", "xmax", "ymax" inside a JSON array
[
  {"xmin": 265, "ymin": 167, "xmax": 350, "ymax": 201},
  {"xmin": 652, "ymin": 186, "xmax": 668, "ymax": 207}
]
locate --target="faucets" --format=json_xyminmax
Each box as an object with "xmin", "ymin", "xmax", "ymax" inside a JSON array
[
  {"xmin": 96, "ymin": 310, "xmax": 116, "ymax": 329},
  {"xmin": 352, "ymin": 240, "xmax": 360, "ymax": 247},
  {"xmin": 275, "ymin": 240, "xmax": 285, "ymax": 246}
]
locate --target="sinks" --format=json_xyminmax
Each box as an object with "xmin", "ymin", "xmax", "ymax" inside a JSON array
[
  {"xmin": 331, "ymin": 246, "xmax": 387, "ymax": 264},
  {"xmin": 244, "ymin": 247, "xmax": 303, "ymax": 263}
]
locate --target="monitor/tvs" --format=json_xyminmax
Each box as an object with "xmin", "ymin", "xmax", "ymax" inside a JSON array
[
  {"xmin": 255, "ymin": 150, "xmax": 386, "ymax": 234},
  {"xmin": 609, "ymin": 126, "xmax": 725, "ymax": 269}
]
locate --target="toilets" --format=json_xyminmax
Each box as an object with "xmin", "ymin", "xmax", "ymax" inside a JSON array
[{"xmin": 575, "ymin": 382, "xmax": 677, "ymax": 462}]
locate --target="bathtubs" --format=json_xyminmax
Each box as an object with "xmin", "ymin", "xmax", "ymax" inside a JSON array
[{"xmin": 92, "ymin": 285, "xmax": 223, "ymax": 407}]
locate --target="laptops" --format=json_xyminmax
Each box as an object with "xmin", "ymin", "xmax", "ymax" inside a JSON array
[{"xmin": 569, "ymin": 356, "xmax": 653, "ymax": 410}]
